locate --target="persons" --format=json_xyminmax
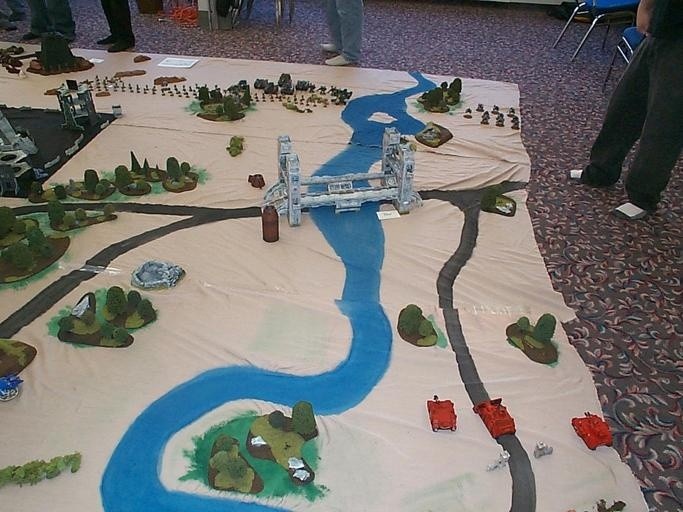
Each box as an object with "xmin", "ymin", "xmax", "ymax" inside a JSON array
[
  {"xmin": 319, "ymin": 0, "xmax": 362, "ymax": 66},
  {"xmin": 95, "ymin": 1, "xmax": 135, "ymax": 52},
  {"xmin": 22, "ymin": 1, "xmax": 75, "ymax": 44},
  {"xmin": 563, "ymin": 1, "xmax": 682, "ymax": 220}
]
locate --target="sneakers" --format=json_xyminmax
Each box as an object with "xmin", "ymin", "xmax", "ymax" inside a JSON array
[
  {"xmin": 22, "ymin": 30, "xmax": 39, "ymax": 41},
  {"xmin": 97, "ymin": 34, "xmax": 135, "ymax": 54}
]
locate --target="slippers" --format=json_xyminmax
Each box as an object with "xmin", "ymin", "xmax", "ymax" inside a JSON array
[
  {"xmin": 567, "ymin": 168, "xmax": 585, "ymax": 187},
  {"xmin": 610, "ymin": 203, "xmax": 649, "ymax": 223}
]
